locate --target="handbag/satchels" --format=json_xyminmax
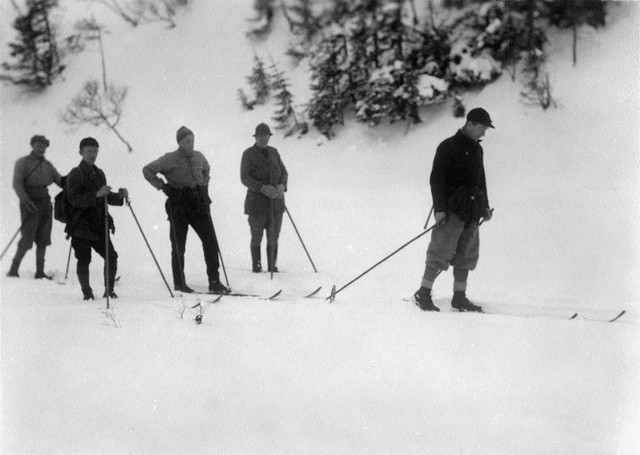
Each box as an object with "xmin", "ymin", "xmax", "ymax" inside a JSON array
[{"xmin": 22, "ymin": 159, "xmax": 42, "ymax": 182}]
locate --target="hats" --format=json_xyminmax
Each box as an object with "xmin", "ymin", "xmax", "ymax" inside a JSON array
[
  {"xmin": 176, "ymin": 126, "xmax": 194, "ymax": 143},
  {"xmin": 253, "ymin": 123, "xmax": 273, "ymax": 137},
  {"xmin": 467, "ymin": 108, "xmax": 495, "ymax": 129}
]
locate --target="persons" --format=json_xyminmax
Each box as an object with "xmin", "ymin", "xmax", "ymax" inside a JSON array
[
  {"xmin": 414, "ymin": 108, "xmax": 496, "ymax": 313},
  {"xmin": 239, "ymin": 122, "xmax": 289, "ymax": 272},
  {"xmin": 64, "ymin": 137, "xmax": 128, "ymax": 300},
  {"xmin": 7, "ymin": 134, "xmax": 61, "ymax": 280},
  {"xmin": 142, "ymin": 125, "xmax": 230, "ymax": 293}
]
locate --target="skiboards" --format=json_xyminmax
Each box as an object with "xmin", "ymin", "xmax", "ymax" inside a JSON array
[
  {"xmin": 190, "ymin": 287, "xmax": 323, "ymax": 300},
  {"xmin": 399, "ymin": 297, "xmax": 626, "ymax": 323},
  {"xmin": 192, "ymin": 294, "xmax": 222, "ymax": 308}
]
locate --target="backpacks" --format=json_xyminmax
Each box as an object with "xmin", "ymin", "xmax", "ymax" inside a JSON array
[{"xmin": 54, "ymin": 176, "xmax": 71, "ymax": 222}]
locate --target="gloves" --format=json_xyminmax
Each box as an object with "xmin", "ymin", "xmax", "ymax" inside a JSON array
[
  {"xmin": 274, "ymin": 184, "xmax": 286, "ymax": 200},
  {"xmin": 261, "ymin": 185, "xmax": 279, "ymax": 200}
]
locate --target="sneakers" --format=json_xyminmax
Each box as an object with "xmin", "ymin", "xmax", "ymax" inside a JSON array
[
  {"xmin": 252, "ymin": 268, "xmax": 266, "ymax": 275},
  {"xmin": 102, "ymin": 292, "xmax": 119, "ymax": 300},
  {"xmin": 7, "ymin": 270, "xmax": 21, "ymax": 279},
  {"xmin": 34, "ymin": 273, "xmax": 53, "ymax": 281},
  {"xmin": 268, "ymin": 267, "xmax": 280, "ymax": 273},
  {"xmin": 208, "ymin": 280, "xmax": 231, "ymax": 295},
  {"xmin": 82, "ymin": 294, "xmax": 95, "ymax": 302},
  {"xmin": 415, "ymin": 286, "xmax": 440, "ymax": 312},
  {"xmin": 174, "ymin": 282, "xmax": 194, "ymax": 293},
  {"xmin": 451, "ymin": 290, "xmax": 481, "ymax": 312}
]
